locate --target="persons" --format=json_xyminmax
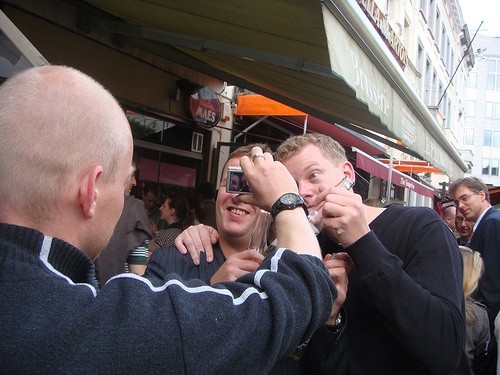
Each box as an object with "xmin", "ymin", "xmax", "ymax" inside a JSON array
[
  {"xmin": 441, "ymin": 175, "xmax": 500, "ymax": 375},
  {"xmin": 174, "ymin": 131, "xmax": 466, "ymax": 375},
  {"xmin": 0, "ymin": 63, "xmax": 338, "ymax": 375},
  {"xmin": 229, "ymin": 173, "xmax": 252, "ymax": 193},
  {"xmin": 92, "ymin": 160, "xmax": 216, "ymax": 289},
  {"xmin": 141, "ymin": 144, "xmax": 354, "ymax": 375}
]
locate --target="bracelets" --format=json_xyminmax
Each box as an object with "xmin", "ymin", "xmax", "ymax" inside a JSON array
[{"xmin": 336, "ymin": 313, "xmax": 341, "ymax": 325}]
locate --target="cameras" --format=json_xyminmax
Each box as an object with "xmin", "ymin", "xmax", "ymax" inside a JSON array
[{"xmin": 226, "ymin": 167, "xmax": 253, "ymax": 195}]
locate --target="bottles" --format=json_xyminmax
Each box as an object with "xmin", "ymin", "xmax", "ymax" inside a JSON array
[{"xmin": 261, "ymin": 177, "xmax": 354, "ymax": 258}]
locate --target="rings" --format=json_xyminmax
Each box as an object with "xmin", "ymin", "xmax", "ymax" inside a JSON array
[{"xmin": 252, "ymin": 154, "xmax": 265, "ymax": 162}]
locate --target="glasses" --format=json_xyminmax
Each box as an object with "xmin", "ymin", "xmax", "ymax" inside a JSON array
[
  {"xmin": 453, "ymin": 215, "xmax": 473, "ymax": 224},
  {"xmin": 452, "ymin": 192, "xmax": 478, "ymax": 207}
]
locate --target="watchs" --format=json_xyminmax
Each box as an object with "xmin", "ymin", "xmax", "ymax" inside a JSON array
[{"xmin": 271, "ymin": 193, "xmax": 309, "ymax": 219}]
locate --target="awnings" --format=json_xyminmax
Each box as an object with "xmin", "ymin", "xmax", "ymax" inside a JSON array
[
  {"xmin": 87, "ymin": 0, "xmax": 470, "ymax": 181},
  {"xmin": 236, "ymin": 91, "xmax": 434, "ymax": 199}
]
